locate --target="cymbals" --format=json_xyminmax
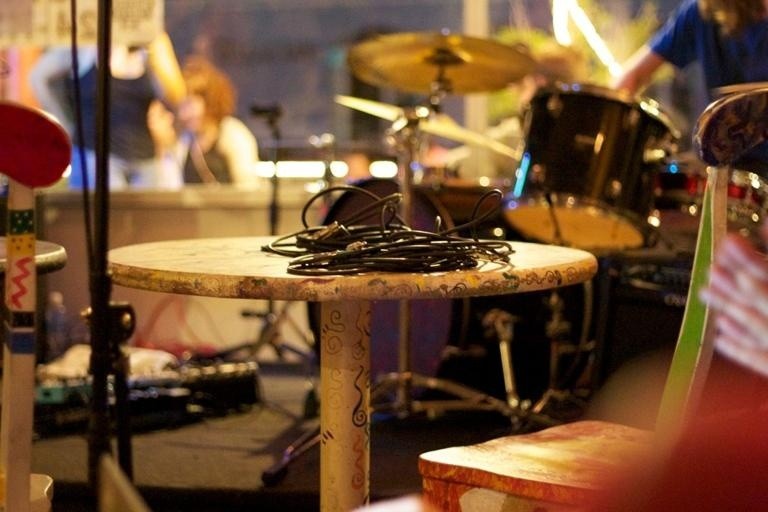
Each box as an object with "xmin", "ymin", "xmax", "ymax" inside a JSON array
[
  {"xmin": 349, "ymin": 31, "xmax": 540, "ymax": 96},
  {"xmin": 334, "ymin": 94, "xmax": 522, "ymax": 161}
]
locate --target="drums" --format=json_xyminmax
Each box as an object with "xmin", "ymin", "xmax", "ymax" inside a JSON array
[
  {"xmin": 305, "ymin": 177, "xmax": 593, "ymax": 394},
  {"xmin": 501, "ymin": 78, "xmax": 679, "ymax": 255}
]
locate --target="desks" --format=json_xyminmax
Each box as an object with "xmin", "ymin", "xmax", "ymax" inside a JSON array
[
  {"xmin": 107, "ymin": 233, "xmax": 598, "ymax": 512},
  {"xmin": 0, "ymin": 238, "xmax": 67, "ymax": 356}
]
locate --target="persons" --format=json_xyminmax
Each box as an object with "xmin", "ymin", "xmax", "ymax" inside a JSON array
[
  {"xmin": 28, "ymin": 1, "xmax": 189, "ymax": 188},
  {"xmin": 146, "ymin": 69, "xmax": 259, "ymax": 186},
  {"xmin": 612, "ymin": 3, "xmax": 766, "ymax": 95}
]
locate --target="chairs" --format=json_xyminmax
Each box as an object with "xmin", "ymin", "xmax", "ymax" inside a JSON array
[
  {"xmin": 416, "ymin": 86, "xmax": 766, "ymax": 512},
  {"xmin": 0, "ymin": 97, "xmax": 71, "ymax": 511}
]
